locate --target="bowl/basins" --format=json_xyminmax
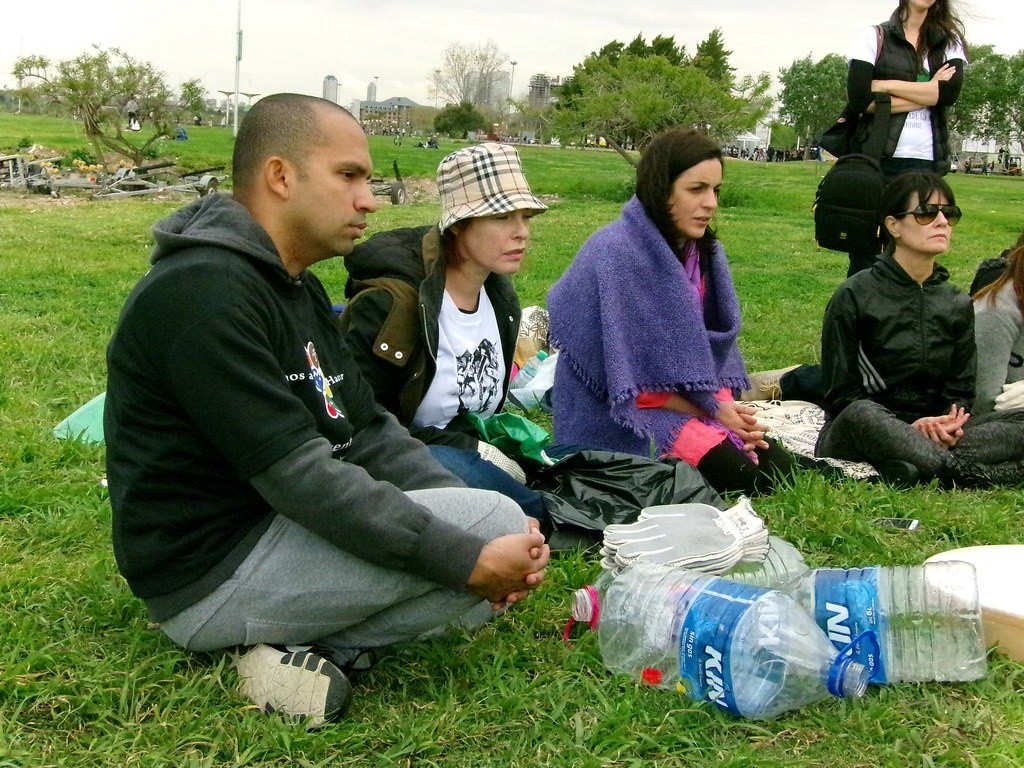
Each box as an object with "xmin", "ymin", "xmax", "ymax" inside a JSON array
[{"xmin": 917, "ymin": 545, "xmax": 1024, "ymax": 666}]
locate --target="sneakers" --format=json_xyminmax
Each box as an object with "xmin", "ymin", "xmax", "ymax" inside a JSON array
[{"xmin": 737, "ymin": 364, "xmax": 802, "ymax": 400}]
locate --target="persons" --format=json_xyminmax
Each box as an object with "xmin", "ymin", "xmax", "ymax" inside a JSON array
[
  {"xmin": 193, "ymin": 114, "xmax": 202, "ymax": 126},
  {"xmin": 846, "ymin": 0, "xmax": 970, "ymax": 278},
  {"xmin": 427, "ymin": 135, "xmax": 439, "ymax": 148},
  {"xmin": 125, "ymin": 97, "xmax": 137, "ymax": 129},
  {"xmin": 509, "ymin": 135, "xmax": 539, "ymax": 144},
  {"xmin": 815, "ymin": 170, "xmax": 1024, "ymax": 491},
  {"xmin": 361, "ymin": 127, "xmax": 374, "ymax": 135},
  {"xmin": 950, "ymin": 147, "xmax": 1011, "ymax": 175},
  {"xmin": 968, "ymin": 234, "xmax": 1024, "ymax": 412},
  {"xmin": 548, "ymin": 132, "xmax": 796, "ymax": 499},
  {"xmin": 103, "ymin": 93, "xmax": 551, "ymax": 730},
  {"xmin": 383, "ymin": 126, "xmax": 406, "ymax": 136},
  {"xmin": 174, "ymin": 124, "xmax": 188, "ymax": 140},
  {"xmin": 722, "ymin": 144, "xmax": 820, "ymax": 162},
  {"xmin": 339, "ymin": 144, "xmax": 554, "ymax": 541}
]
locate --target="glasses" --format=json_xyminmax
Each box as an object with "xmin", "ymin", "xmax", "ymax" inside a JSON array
[{"xmin": 898, "ymin": 203, "xmax": 962, "ymax": 226}]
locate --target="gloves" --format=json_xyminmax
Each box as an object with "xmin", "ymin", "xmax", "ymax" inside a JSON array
[
  {"xmin": 599, "ymin": 499, "xmax": 770, "ymax": 579},
  {"xmin": 475, "ymin": 440, "xmax": 526, "ymax": 483}
]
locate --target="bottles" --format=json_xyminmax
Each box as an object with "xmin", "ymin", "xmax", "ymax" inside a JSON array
[
  {"xmin": 563, "ymin": 533, "xmax": 804, "ymax": 653},
  {"xmin": 757, "ymin": 565, "xmax": 988, "ymax": 684},
  {"xmin": 507, "ymin": 349, "xmax": 549, "ymax": 395},
  {"xmin": 595, "ymin": 562, "xmax": 870, "ymax": 720}
]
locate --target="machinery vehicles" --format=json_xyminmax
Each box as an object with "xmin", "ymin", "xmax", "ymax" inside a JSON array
[{"xmin": 1007, "ymin": 157, "xmax": 1023, "ymax": 176}]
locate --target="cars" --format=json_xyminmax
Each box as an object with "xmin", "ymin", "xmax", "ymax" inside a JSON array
[
  {"xmin": 966, "ymin": 155, "xmax": 983, "ymax": 167},
  {"xmin": 973, "ymin": 163, "xmax": 991, "ymax": 173}
]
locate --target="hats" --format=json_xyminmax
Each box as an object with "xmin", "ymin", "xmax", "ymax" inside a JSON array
[{"xmin": 435, "ymin": 143, "xmax": 548, "ymax": 237}]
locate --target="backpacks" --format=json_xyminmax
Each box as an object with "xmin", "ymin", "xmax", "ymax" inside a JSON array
[{"xmin": 814, "ymin": 94, "xmax": 891, "ymax": 254}]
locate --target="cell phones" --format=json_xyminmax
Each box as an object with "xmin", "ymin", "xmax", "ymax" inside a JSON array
[{"xmin": 872, "ymin": 517, "xmax": 919, "ymax": 531}]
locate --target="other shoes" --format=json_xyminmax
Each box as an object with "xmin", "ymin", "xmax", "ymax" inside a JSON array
[
  {"xmin": 237, "ymin": 644, "xmax": 352, "ymax": 731},
  {"xmin": 874, "ymin": 457, "xmax": 919, "ymax": 488}
]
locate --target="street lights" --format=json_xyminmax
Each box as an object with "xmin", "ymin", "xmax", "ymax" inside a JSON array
[
  {"xmin": 509, "ymin": 61, "xmax": 518, "ymax": 112},
  {"xmin": 435, "ymin": 71, "xmax": 441, "ymax": 109}
]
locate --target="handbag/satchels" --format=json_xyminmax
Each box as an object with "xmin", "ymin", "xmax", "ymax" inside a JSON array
[{"xmin": 465, "ymin": 412, "xmax": 730, "ymax": 560}]
